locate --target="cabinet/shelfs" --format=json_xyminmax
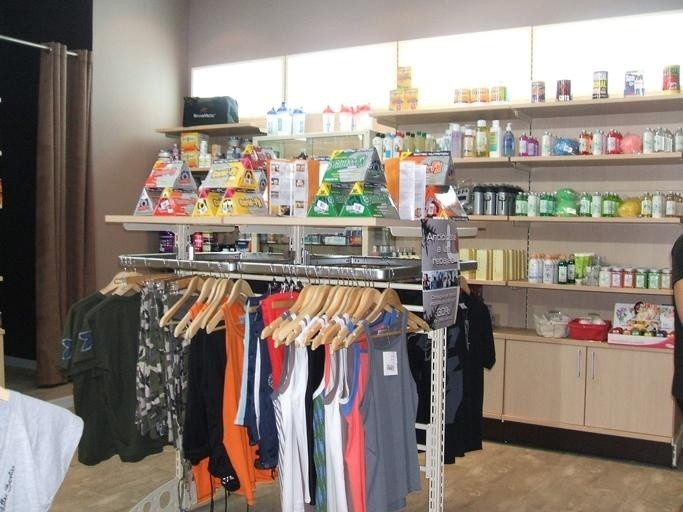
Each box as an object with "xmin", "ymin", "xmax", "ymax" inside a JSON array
[{"xmin": 367, "ymin": 87, "xmax": 683, "ymax": 469}]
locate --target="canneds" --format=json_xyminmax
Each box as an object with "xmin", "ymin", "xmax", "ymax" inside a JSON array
[
  {"xmin": 556, "ymin": 80, "xmax": 571, "ymax": 101},
  {"xmin": 599, "ymin": 266, "xmax": 672, "ymax": 289},
  {"xmin": 624, "ymin": 71, "xmax": 645, "ymax": 97},
  {"xmin": 530, "ymin": 81, "xmax": 545, "ymax": 103},
  {"xmin": 662, "ymin": 64, "xmax": 680, "ymax": 95},
  {"xmin": 592, "ymin": 71, "xmax": 608, "ymax": 99}
]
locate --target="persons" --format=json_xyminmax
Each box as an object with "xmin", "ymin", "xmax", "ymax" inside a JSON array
[{"xmin": 669, "ymin": 236, "xmax": 682, "ymax": 467}]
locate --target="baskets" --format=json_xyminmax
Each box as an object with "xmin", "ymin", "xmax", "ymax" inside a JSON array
[{"xmin": 533, "ymin": 311, "xmax": 572, "ymax": 338}]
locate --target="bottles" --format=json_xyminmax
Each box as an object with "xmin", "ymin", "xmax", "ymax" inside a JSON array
[
  {"xmin": 472, "ymin": 184, "xmax": 683, "ymax": 218},
  {"xmin": 526, "ymin": 253, "xmax": 575, "ymax": 286},
  {"xmin": 198, "ymin": 140, "xmax": 211, "ymax": 169},
  {"xmin": 373, "ymin": 118, "xmax": 683, "ymax": 158},
  {"xmin": 172, "ymin": 143, "xmax": 179, "ymax": 161},
  {"xmin": 599, "ymin": 264, "xmax": 673, "ymax": 290},
  {"xmin": 266, "ymin": 102, "xmax": 306, "ymax": 136},
  {"xmin": 661, "ymin": 65, "xmax": 680, "ymax": 91},
  {"xmin": 320, "ymin": 105, "xmax": 374, "ymax": 134}
]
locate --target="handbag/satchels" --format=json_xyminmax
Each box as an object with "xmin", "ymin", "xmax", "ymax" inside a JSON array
[{"xmin": 183, "ymin": 97, "xmax": 239, "ymax": 127}]
